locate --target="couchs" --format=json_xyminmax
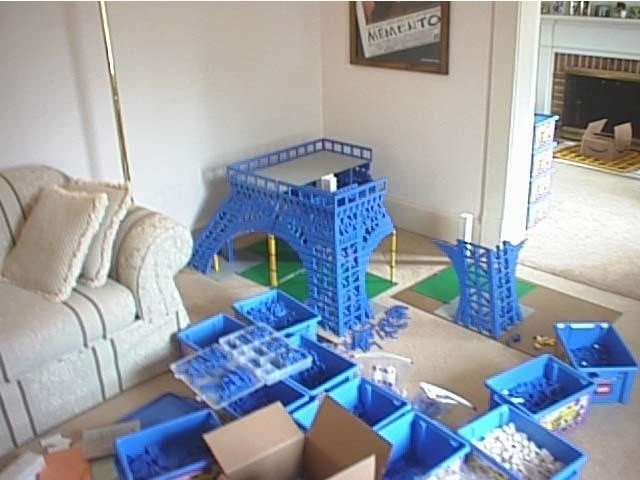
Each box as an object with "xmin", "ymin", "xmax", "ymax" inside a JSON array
[{"xmin": 0, "ymin": 163, "xmax": 196, "ymax": 463}]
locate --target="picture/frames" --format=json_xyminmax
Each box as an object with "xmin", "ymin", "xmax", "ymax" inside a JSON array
[{"xmin": 347, "ymin": 1, "xmax": 451, "ymax": 77}]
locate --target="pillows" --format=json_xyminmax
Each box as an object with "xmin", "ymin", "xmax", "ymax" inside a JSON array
[
  {"xmin": 0, "ymin": 181, "xmax": 111, "ymax": 304},
  {"xmin": 64, "ymin": 173, "xmax": 135, "ymax": 291}
]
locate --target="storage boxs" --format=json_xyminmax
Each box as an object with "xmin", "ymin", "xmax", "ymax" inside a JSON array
[
  {"xmin": 231, "ymin": 290, "xmax": 323, "ymax": 351},
  {"xmin": 178, "ymin": 313, "xmax": 247, "ymax": 367},
  {"xmin": 363, "ymin": 407, "xmax": 471, "ymax": 477},
  {"xmin": 290, "ymin": 378, "xmax": 409, "ymax": 443},
  {"xmin": 114, "ymin": 409, "xmax": 227, "ymax": 479},
  {"xmin": 580, "ymin": 117, "xmax": 633, "ymax": 162},
  {"xmin": 457, "ymin": 401, "xmax": 588, "ymax": 478},
  {"xmin": 483, "ymin": 351, "xmax": 597, "ymax": 437},
  {"xmin": 260, "ymin": 334, "xmax": 359, "ymax": 396},
  {"xmin": 227, "ymin": 379, "xmax": 308, "ymax": 426},
  {"xmin": 552, "ymin": 318, "xmax": 640, "ymax": 407},
  {"xmin": 200, "ymin": 394, "xmax": 394, "ymax": 477}
]
